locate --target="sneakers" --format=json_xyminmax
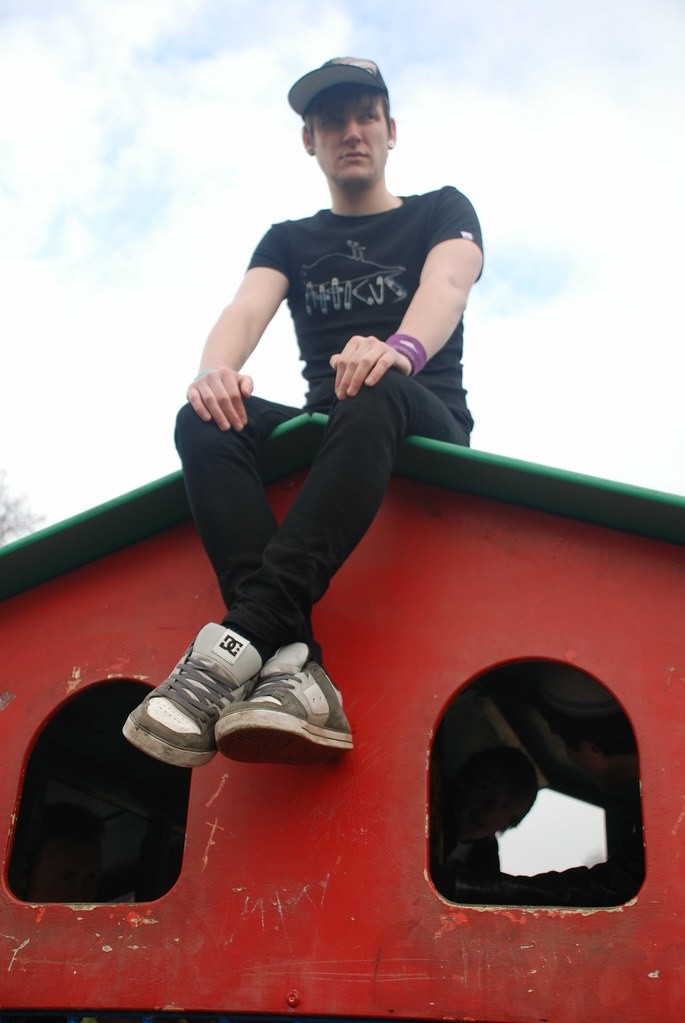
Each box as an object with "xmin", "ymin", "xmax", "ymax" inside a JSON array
[
  {"xmin": 121, "ymin": 622, "xmax": 263, "ymax": 767},
  {"xmin": 214, "ymin": 642, "xmax": 354, "ymax": 764}
]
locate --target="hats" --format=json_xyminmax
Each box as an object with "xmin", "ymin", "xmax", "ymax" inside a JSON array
[{"xmin": 287, "ymin": 57, "xmax": 390, "ymax": 118}]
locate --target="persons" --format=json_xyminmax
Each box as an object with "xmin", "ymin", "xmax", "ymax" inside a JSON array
[{"xmin": 120, "ymin": 56, "xmax": 484, "ymax": 766}]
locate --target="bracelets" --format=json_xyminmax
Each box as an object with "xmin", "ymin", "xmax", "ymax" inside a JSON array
[{"xmin": 385, "ymin": 334, "xmax": 427, "ymax": 377}]
